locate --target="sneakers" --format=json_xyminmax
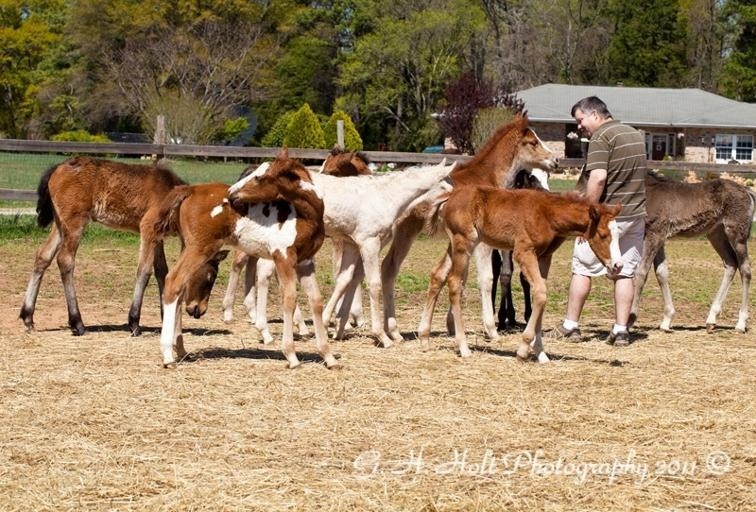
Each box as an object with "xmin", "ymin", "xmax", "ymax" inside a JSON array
[
  {"xmin": 543, "ymin": 325, "xmax": 581, "ymax": 343},
  {"xmin": 605, "ymin": 328, "xmax": 630, "ymax": 347}
]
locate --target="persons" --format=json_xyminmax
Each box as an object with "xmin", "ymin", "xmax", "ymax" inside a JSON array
[{"xmin": 542, "ymin": 97, "xmax": 647, "ymax": 347}]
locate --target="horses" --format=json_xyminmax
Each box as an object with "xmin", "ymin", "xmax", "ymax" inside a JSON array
[
  {"xmin": 140, "ymin": 146, "xmax": 342, "ymax": 371},
  {"xmin": 221, "ymin": 99, "xmax": 624, "ymax": 365},
  {"xmin": 572, "ymin": 160, "xmax": 756, "ymax": 335},
  {"xmin": 15, "ymin": 152, "xmax": 233, "ymax": 335}
]
